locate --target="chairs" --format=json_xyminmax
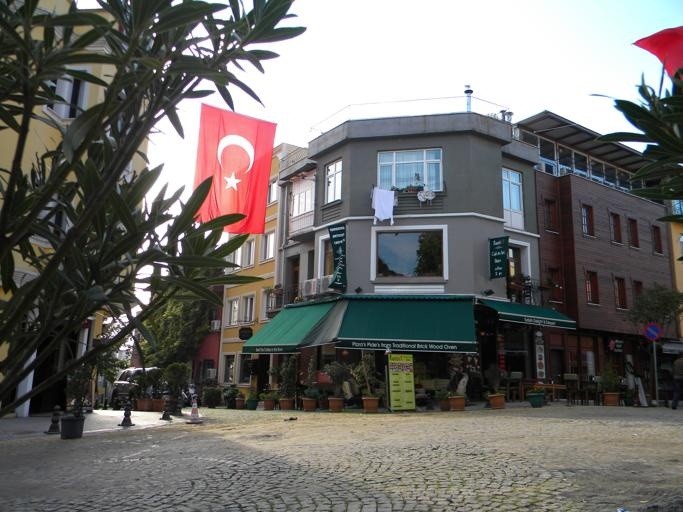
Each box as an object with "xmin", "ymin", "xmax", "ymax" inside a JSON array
[{"xmin": 565, "ymin": 379, "xmax": 583, "ymax": 406}]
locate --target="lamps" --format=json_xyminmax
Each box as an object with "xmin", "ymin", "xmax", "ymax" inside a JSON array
[
  {"xmin": 486, "ymin": 289, "xmax": 494, "ymax": 296},
  {"xmin": 355, "ymin": 286, "xmax": 362, "ymax": 293}
]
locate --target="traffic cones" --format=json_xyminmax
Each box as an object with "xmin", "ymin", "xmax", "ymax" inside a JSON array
[{"xmin": 184, "ymin": 397, "xmax": 201, "ymax": 425}]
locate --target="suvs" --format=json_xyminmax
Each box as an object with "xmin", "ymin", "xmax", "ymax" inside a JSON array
[{"xmin": 108, "ymin": 366, "xmax": 163, "ymax": 409}]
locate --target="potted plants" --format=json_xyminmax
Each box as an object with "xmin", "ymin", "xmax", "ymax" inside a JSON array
[
  {"xmin": 598, "ymin": 360, "xmax": 619, "ymax": 406},
  {"xmin": 318, "ymin": 387, "xmax": 329, "ymax": 410},
  {"xmin": 352, "ymin": 355, "xmax": 385, "ymax": 413},
  {"xmin": 447, "ymin": 392, "xmax": 465, "ymax": 411},
  {"xmin": 326, "ymin": 361, "xmax": 351, "ymax": 411},
  {"xmin": 486, "ymin": 392, "xmax": 505, "ymax": 408},
  {"xmin": 131, "ymin": 363, "xmax": 294, "ymax": 411},
  {"xmin": 435, "ymin": 388, "xmax": 451, "ymax": 410},
  {"xmin": 300, "ymin": 386, "xmax": 318, "ymax": 411},
  {"xmin": 525, "ymin": 387, "xmax": 545, "ymax": 408}
]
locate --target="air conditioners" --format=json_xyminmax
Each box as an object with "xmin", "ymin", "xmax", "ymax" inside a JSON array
[
  {"xmin": 211, "ymin": 320, "xmax": 220, "ymax": 330},
  {"xmin": 301, "ymin": 274, "xmax": 341, "ymax": 296}
]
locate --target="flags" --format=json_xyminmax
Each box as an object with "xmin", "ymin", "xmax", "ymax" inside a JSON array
[
  {"xmin": 633, "ymin": 24, "xmax": 682, "ymax": 85},
  {"xmin": 191, "ymin": 103, "xmax": 276, "ymax": 238}
]
